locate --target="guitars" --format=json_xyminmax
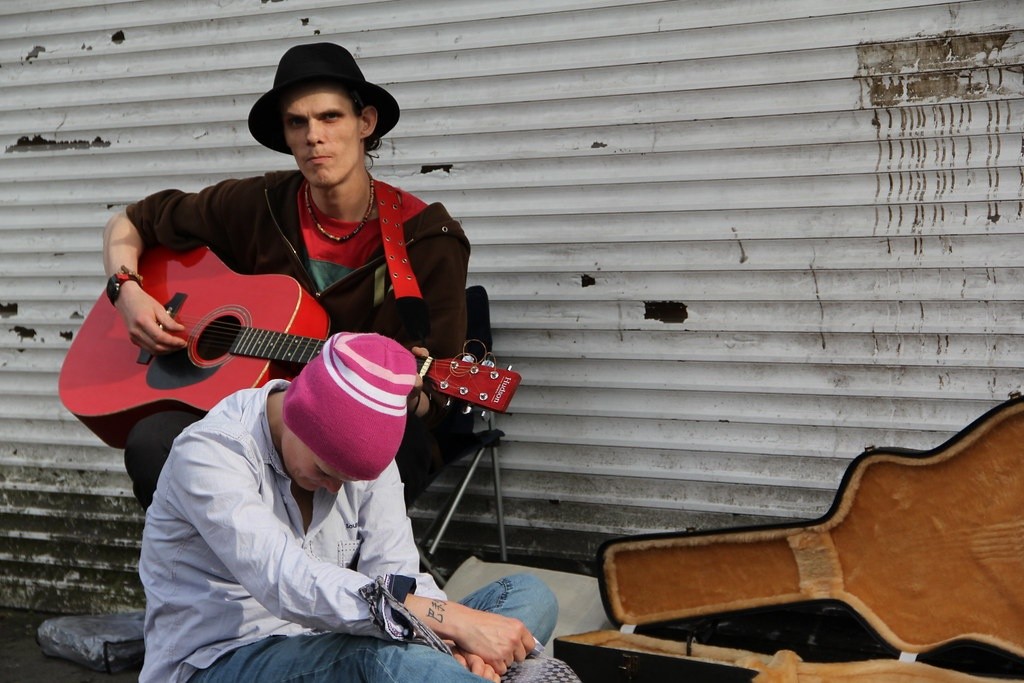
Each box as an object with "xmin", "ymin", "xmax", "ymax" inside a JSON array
[{"xmin": 57, "ymin": 244, "xmax": 522, "ymax": 448}]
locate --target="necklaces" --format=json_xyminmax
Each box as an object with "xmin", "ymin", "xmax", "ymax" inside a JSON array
[{"xmin": 305, "ymin": 169, "xmax": 375, "ymax": 242}]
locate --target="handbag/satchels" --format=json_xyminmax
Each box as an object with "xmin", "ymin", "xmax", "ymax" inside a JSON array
[{"xmin": 359, "ymin": 572, "xmax": 581, "ymax": 683}]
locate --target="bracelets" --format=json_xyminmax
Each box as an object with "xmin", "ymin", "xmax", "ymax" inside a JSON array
[{"xmin": 411, "ymin": 394, "xmax": 420, "ymax": 415}]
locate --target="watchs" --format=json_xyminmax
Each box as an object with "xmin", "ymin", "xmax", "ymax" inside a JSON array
[{"xmin": 106, "ymin": 265, "xmax": 143, "ymax": 308}]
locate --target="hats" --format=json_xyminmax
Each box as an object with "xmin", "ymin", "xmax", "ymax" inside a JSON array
[
  {"xmin": 283, "ymin": 332, "xmax": 417, "ymax": 481},
  {"xmin": 247, "ymin": 42, "xmax": 401, "ymax": 155}
]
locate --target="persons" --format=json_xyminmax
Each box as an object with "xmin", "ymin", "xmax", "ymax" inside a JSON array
[
  {"xmin": 103, "ymin": 42, "xmax": 471, "ymax": 505},
  {"xmin": 134, "ymin": 333, "xmax": 560, "ymax": 683}
]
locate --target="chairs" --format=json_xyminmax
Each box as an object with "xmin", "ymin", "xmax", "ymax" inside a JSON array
[{"xmin": 405, "ymin": 285, "xmax": 510, "ymax": 589}]
